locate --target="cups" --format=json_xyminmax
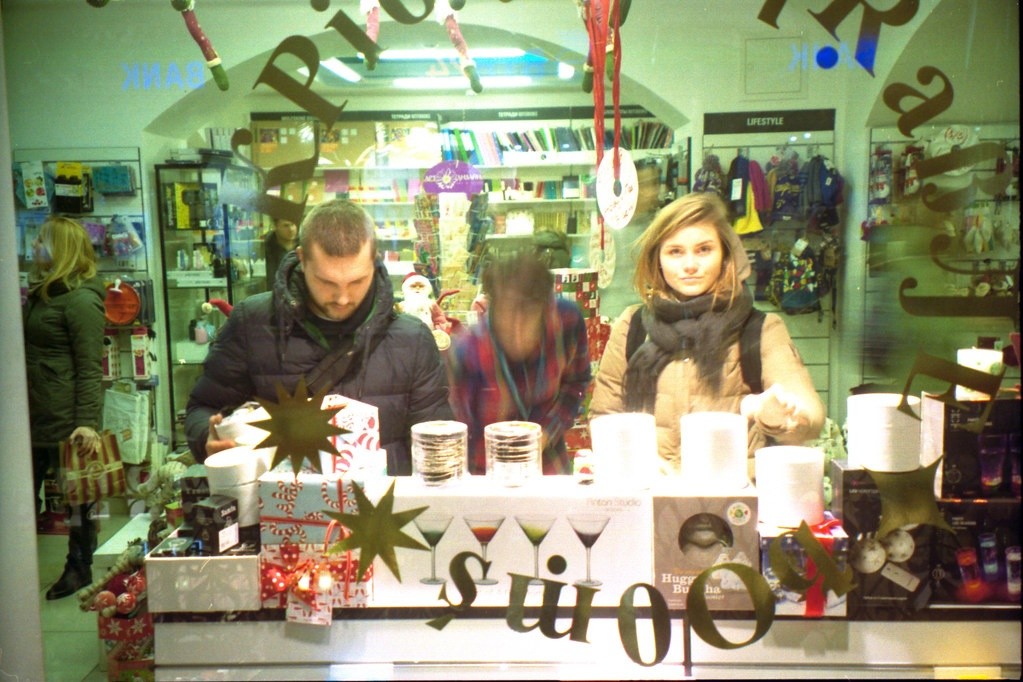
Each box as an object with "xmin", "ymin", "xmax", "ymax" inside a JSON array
[
  {"xmin": 956, "ymin": 533, "xmax": 1021, "ymax": 594},
  {"xmin": 979, "ymin": 432, "xmax": 1023, "ymax": 485}
]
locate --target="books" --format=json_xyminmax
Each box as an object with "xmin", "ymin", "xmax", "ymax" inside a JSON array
[{"xmin": 441, "ymin": 120, "xmax": 672, "ymax": 232}]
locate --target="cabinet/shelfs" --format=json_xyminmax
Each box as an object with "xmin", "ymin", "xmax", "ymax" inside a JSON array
[
  {"xmin": 153, "ymin": 159, "xmax": 270, "ymax": 453},
  {"xmin": 249, "ymin": 104, "xmax": 679, "ymax": 378}
]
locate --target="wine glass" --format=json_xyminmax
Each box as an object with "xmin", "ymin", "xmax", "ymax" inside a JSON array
[
  {"xmin": 464, "ymin": 512, "xmax": 506, "ymax": 585},
  {"xmin": 414, "ymin": 514, "xmax": 453, "ymax": 585},
  {"xmin": 568, "ymin": 514, "xmax": 611, "ymax": 586},
  {"xmin": 513, "ymin": 514, "xmax": 556, "ymax": 586}
]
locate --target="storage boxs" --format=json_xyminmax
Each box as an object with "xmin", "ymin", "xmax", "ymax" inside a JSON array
[
  {"xmin": 931, "ymin": 498, "xmax": 1023, "ymax": 621},
  {"xmin": 260, "ymin": 539, "xmax": 373, "ymax": 608},
  {"xmin": 258, "ymin": 447, "xmax": 388, "ymax": 544},
  {"xmin": 920, "ymin": 393, "xmax": 1023, "ymax": 498},
  {"xmin": 830, "ymin": 459, "xmax": 931, "ymax": 619},
  {"xmin": 760, "ymin": 511, "xmax": 849, "ymax": 616},
  {"xmin": 191, "ymin": 495, "xmax": 239, "ymax": 556}
]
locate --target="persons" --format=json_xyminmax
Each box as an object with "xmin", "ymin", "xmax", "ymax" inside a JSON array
[
  {"xmin": 265, "ymin": 217, "xmax": 300, "ymax": 290},
  {"xmin": 450, "ymin": 256, "xmax": 592, "ymax": 475},
  {"xmin": 184, "ymin": 198, "xmax": 455, "ymax": 476},
  {"xmin": 587, "ymin": 188, "xmax": 825, "ymax": 482},
  {"xmin": 395, "ymin": 273, "xmax": 447, "ymax": 332},
  {"xmin": 22, "ymin": 218, "xmax": 107, "ymax": 600}
]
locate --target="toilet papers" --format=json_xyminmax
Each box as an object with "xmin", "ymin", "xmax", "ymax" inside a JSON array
[
  {"xmin": 754, "ymin": 445, "xmax": 825, "ymax": 529},
  {"xmin": 589, "ymin": 411, "xmax": 656, "ymax": 489},
  {"xmin": 847, "ymin": 393, "xmax": 921, "ymax": 472},
  {"xmin": 679, "ymin": 410, "xmax": 749, "ymax": 492}
]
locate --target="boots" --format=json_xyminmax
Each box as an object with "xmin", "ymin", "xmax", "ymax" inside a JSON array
[{"xmin": 45, "ymin": 525, "xmax": 93, "ymax": 601}]
